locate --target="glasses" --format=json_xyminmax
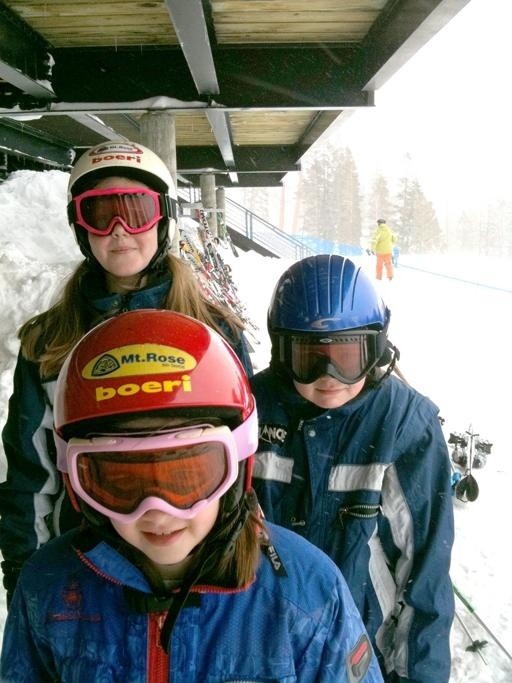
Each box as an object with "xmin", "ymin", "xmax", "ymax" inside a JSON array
[
  {"xmin": 51, "ymin": 392, "xmax": 258, "ymax": 525},
  {"xmin": 66, "ymin": 187, "xmax": 178, "ymax": 237},
  {"xmin": 271, "ymin": 313, "xmax": 391, "ymax": 385}
]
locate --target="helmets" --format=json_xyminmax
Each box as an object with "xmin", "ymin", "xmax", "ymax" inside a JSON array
[
  {"xmin": 266, "ymin": 254, "xmax": 390, "ymax": 334},
  {"xmin": 66, "ymin": 140, "xmax": 178, "ymax": 251},
  {"xmin": 51, "ymin": 306, "xmax": 257, "ymax": 512}
]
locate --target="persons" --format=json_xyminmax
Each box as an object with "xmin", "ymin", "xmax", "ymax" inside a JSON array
[
  {"xmin": 0, "ymin": 309, "xmax": 386, "ymax": 683},
  {"xmin": 248, "ymin": 255, "xmax": 454, "ymax": 683},
  {"xmin": 370, "ymin": 219, "xmax": 397, "ymax": 280},
  {"xmin": 0, "ymin": 141, "xmax": 254, "ymax": 613}
]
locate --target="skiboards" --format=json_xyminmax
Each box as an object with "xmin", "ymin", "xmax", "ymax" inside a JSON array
[
  {"xmin": 179, "ymin": 201, "xmax": 261, "ymax": 353},
  {"xmin": 447, "ymin": 424, "xmax": 493, "ymax": 500}
]
